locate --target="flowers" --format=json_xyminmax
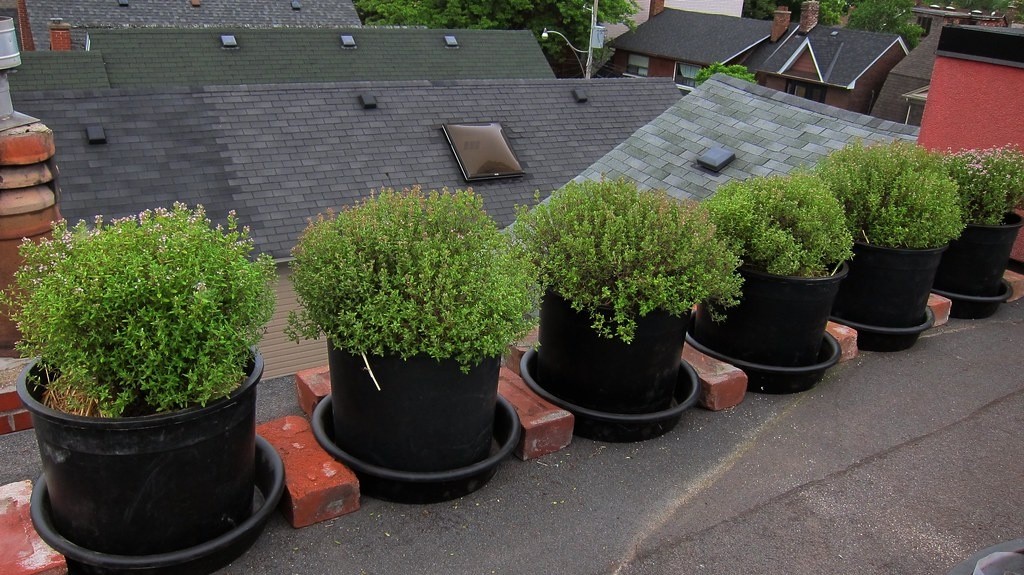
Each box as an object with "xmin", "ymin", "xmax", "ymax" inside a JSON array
[
  {"xmin": 285, "ymin": 184, "xmax": 547, "ymax": 373},
  {"xmin": 0, "ymin": 200, "xmax": 281, "ymax": 420},
  {"xmin": 699, "ymin": 162, "xmax": 855, "ymax": 280},
  {"xmin": 819, "ymin": 134, "xmax": 967, "ymax": 250},
  {"xmin": 507, "ymin": 169, "xmax": 747, "ymax": 344},
  {"xmin": 942, "ymin": 141, "xmax": 1024, "ymax": 227}
]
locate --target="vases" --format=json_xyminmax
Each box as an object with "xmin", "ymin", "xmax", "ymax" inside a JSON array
[
  {"xmin": 682, "ymin": 261, "xmax": 850, "ymax": 396},
  {"xmin": 20, "ymin": 347, "xmax": 285, "ymax": 575},
  {"xmin": 310, "ymin": 334, "xmax": 521, "ymax": 506},
  {"xmin": 931, "ymin": 212, "xmax": 1024, "ymax": 321},
  {"xmin": 520, "ymin": 294, "xmax": 699, "ymax": 446},
  {"xmin": 829, "ymin": 242, "xmax": 950, "ymax": 352}
]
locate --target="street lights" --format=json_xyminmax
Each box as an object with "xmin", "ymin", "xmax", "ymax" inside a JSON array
[{"xmin": 542, "ymin": 0, "xmax": 601, "ymax": 78}]
